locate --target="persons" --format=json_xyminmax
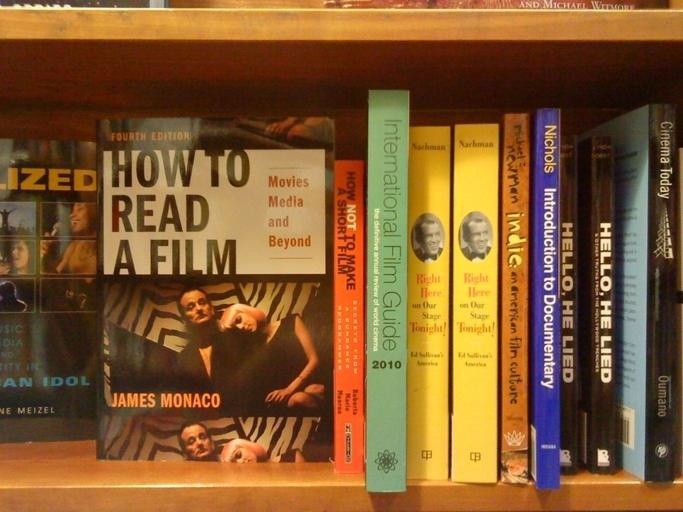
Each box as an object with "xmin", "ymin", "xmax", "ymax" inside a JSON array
[
  {"xmin": 1, "ymin": 237, "xmax": 34, "ymax": 275},
  {"xmin": 39, "ymin": 201, "xmax": 96, "ymax": 312},
  {"xmin": 178, "ymin": 287, "xmax": 334, "ymax": 463}
]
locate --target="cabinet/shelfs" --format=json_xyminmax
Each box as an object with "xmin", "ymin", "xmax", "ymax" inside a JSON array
[{"xmin": 0, "ymin": 0, "xmax": 683, "ymax": 512}]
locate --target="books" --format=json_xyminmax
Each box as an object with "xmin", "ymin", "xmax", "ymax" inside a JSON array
[
  {"xmin": 368, "ymin": 91, "xmax": 683, "ymax": 495},
  {"xmin": 1, "ymin": 137, "xmax": 96, "ymax": 442},
  {"xmin": 95, "ymin": 116, "xmax": 336, "ymax": 463},
  {"xmin": 334, "ymin": 158, "xmax": 366, "ymax": 475}
]
